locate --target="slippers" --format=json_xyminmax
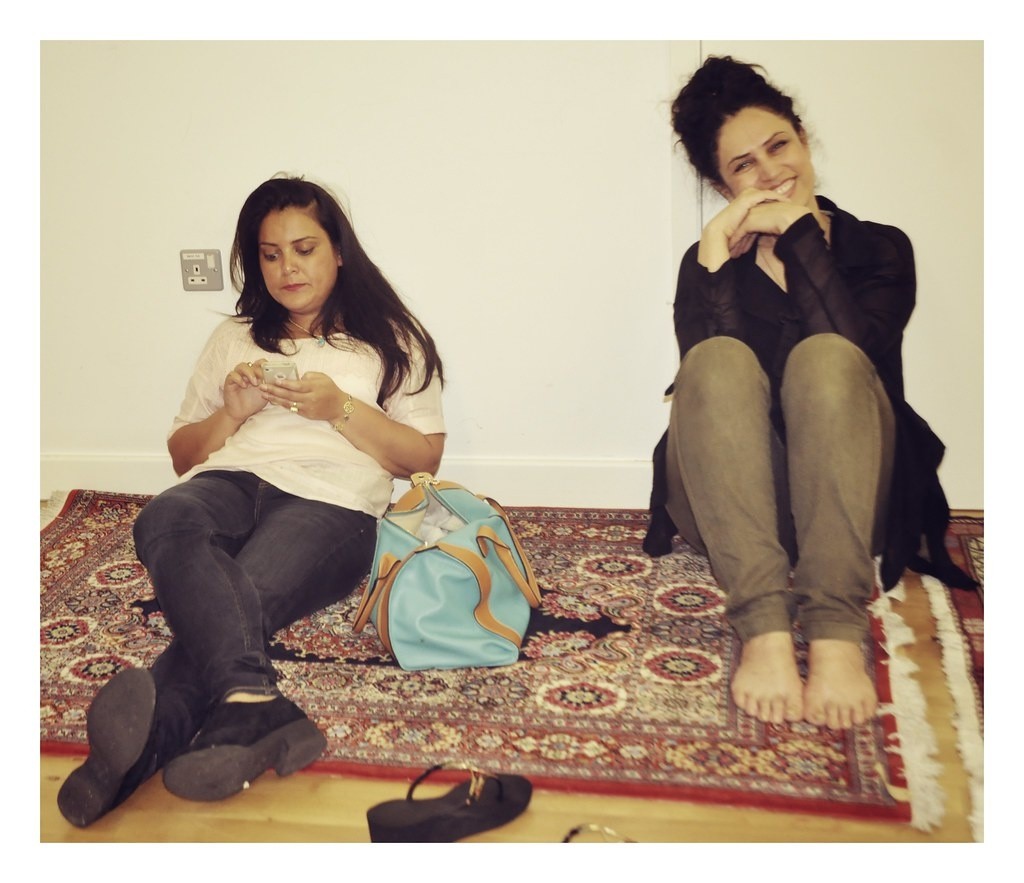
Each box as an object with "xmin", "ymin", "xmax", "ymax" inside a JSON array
[
  {"xmin": 365, "ymin": 760, "xmax": 533, "ymax": 843},
  {"xmin": 562, "ymin": 822, "xmax": 637, "ymax": 843}
]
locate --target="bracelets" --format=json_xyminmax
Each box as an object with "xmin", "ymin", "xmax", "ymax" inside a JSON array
[{"xmin": 333, "ymin": 395, "xmax": 354, "ymax": 431}]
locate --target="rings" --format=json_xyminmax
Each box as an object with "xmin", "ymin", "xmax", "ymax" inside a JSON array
[
  {"xmin": 247, "ymin": 362, "xmax": 253, "ymax": 367},
  {"xmin": 291, "ymin": 402, "xmax": 298, "ymax": 413}
]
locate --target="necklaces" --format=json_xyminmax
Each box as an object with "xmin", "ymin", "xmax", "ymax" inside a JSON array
[
  {"xmin": 759, "ymin": 249, "xmax": 786, "ymax": 289},
  {"xmin": 289, "ymin": 318, "xmax": 340, "ymax": 347}
]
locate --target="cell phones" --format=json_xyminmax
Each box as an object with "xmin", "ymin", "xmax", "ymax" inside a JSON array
[{"xmin": 260, "ymin": 362, "xmax": 301, "ymax": 407}]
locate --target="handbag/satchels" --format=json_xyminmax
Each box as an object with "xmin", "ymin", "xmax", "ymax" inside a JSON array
[{"xmin": 353, "ymin": 471, "xmax": 542, "ymax": 670}]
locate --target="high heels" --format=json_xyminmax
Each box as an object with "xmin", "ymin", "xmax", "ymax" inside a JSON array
[
  {"xmin": 163, "ymin": 692, "xmax": 328, "ymax": 802},
  {"xmin": 56, "ymin": 666, "xmax": 195, "ymax": 828}
]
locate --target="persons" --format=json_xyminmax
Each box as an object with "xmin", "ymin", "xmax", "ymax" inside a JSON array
[
  {"xmin": 644, "ymin": 54, "xmax": 980, "ymax": 729},
  {"xmin": 57, "ymin": 179, "xmax": 447, "ymax": 827}
]
